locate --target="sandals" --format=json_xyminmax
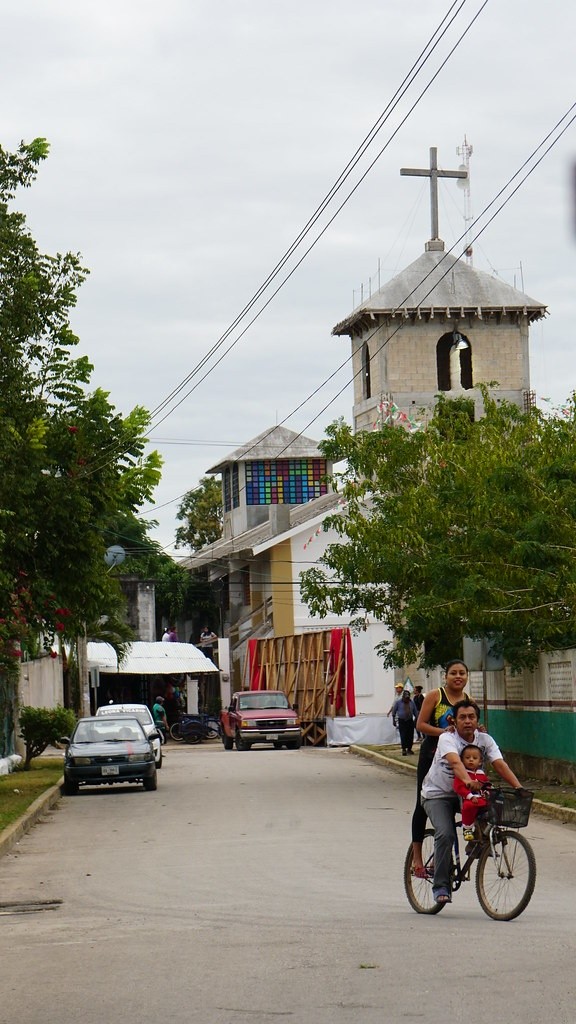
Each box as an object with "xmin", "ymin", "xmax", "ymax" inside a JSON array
[{"xmin": 413, "ymin": 864, "xmax": 434, "ymax": 878}]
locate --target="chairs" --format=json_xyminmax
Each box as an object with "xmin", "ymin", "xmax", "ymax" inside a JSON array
[
  {"xmin": 83, "ymin": 728, "xmax": 133, "ymax": 740},
  {"xmin": 240, "ymin": 700, "xmax": 277, "ymax": 708}
]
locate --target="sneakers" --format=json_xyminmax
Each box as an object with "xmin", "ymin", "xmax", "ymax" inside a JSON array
[{"xmin": 463, "ymin": 827, "xmax": 474, "ymax": 841}]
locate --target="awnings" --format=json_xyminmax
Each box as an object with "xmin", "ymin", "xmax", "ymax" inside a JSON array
[{"xmin": 51, "ymin": 642, "xmax": 219, "ymax": 674}]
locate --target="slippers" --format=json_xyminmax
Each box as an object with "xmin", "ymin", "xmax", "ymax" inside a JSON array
[{"xmin": 434, "ymin": 888, "xmax": 452, "ymax": 903}]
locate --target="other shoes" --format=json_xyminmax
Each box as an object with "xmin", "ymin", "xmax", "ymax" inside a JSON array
[
  {"xmin": 402, "ymin": 750, "xmax": 414, "ymax": 756},
  {"xmin": 466, "ymin": 846, "xmax": 500, "ymax": 857},
  {"xmin": 417, "ymin": 737, "xmax": 423, "ymax": 741}
]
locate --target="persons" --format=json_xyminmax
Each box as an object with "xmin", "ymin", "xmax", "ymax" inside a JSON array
[
  {"xmin": 200, "ymin": 626, "xmax": 217, "ymax": 674},
  {"xmin": 412, "ymin": 660, "xmax": 488, "ymax": 878},
  {"xmin": 454, "ymin": 744, "xmax": 495, "ymax": 840},
  {"xmin": 162, "ymin": 627, "xmax": 178, "ymax": 642},
  {"xmin": 387, "ymin": 683, "xmax": 425, "ymax": 756},
  {"xmin": 421, "ymin": 700, "xmax": 530, "ymax": 905},
  {"xmin": 153, "ymin": 696, "xmax": 169, "ymax": 744}
]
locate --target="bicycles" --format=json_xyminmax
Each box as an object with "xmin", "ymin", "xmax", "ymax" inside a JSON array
[
  {"xmin": 404, "ymin": 779, "xmax": 543, "ymax": 921},
  {"xmin": 170, "ymin": 710, "xmax": 220, "ymax": 743}
]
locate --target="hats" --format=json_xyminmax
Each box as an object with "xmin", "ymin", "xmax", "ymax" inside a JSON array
[
  {"xmin": 395, "ymin": 683, "xmax": 403, "ymax": 687},
  {"xmin": 156, "ymin": 697, "xmax": 164, "ymax": 702}
]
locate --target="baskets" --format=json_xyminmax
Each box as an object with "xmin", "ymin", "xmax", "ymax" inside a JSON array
[{"xmin": 489, "ymin": 787, "xmax": 535, "ymax": 828}]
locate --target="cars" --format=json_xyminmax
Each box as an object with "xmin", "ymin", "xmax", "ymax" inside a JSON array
[
  {"xmin": 94, "ymin": 704, "xmax": 162, "ymax": 769},
  {"xmin": 60, "ymin": 715, "xmax": 160, "ymax": 795}
]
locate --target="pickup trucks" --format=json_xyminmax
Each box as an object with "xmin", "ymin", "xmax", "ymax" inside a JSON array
[{"xmin": 221, "ymin": 691, "xmax": 302, "ymax": 751}]
